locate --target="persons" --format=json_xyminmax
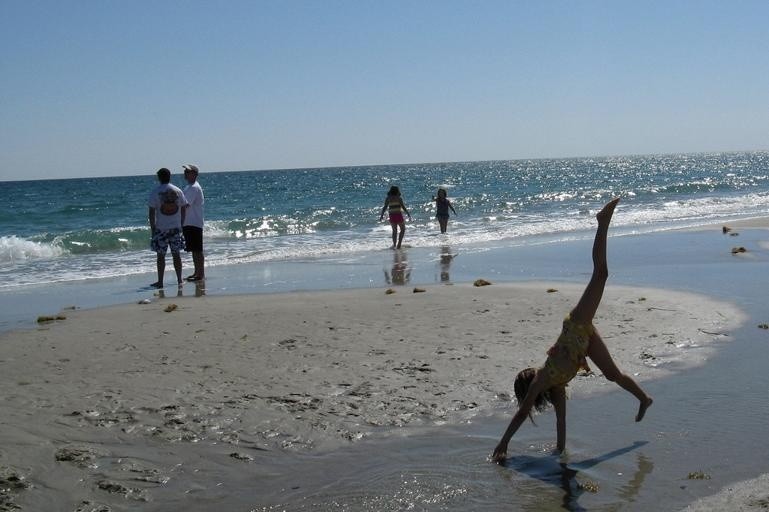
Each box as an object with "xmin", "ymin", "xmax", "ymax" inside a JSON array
[
  {"xmin": 181, "ymin": 164, "xmax": 205, "ymax": 282},
  {"xmin": 492, "ymin": 198, "xmax": 653, "ymax": 460},
  {"xmin": 439, "ymin": 246, "xmax": 458, "ymax": 283},
  {"xmin": 379, "ymin": 186, "xmax": 412, "ymax": 249},
  {"xmin": 148, "ymin": 168, "xmax": 188, "ymax": 288},
  {"xmin": 432, "ymin": 189, "xmax": 458, "ymax": 234},
  {"xmin": 384, "ymin": 249, "xmax": 415, "ymax": 287}
]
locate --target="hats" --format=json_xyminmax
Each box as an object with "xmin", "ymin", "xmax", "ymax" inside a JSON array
[{"xmin": 182, "ymin": 164, "xmax": 199, "ymax": 176}]
